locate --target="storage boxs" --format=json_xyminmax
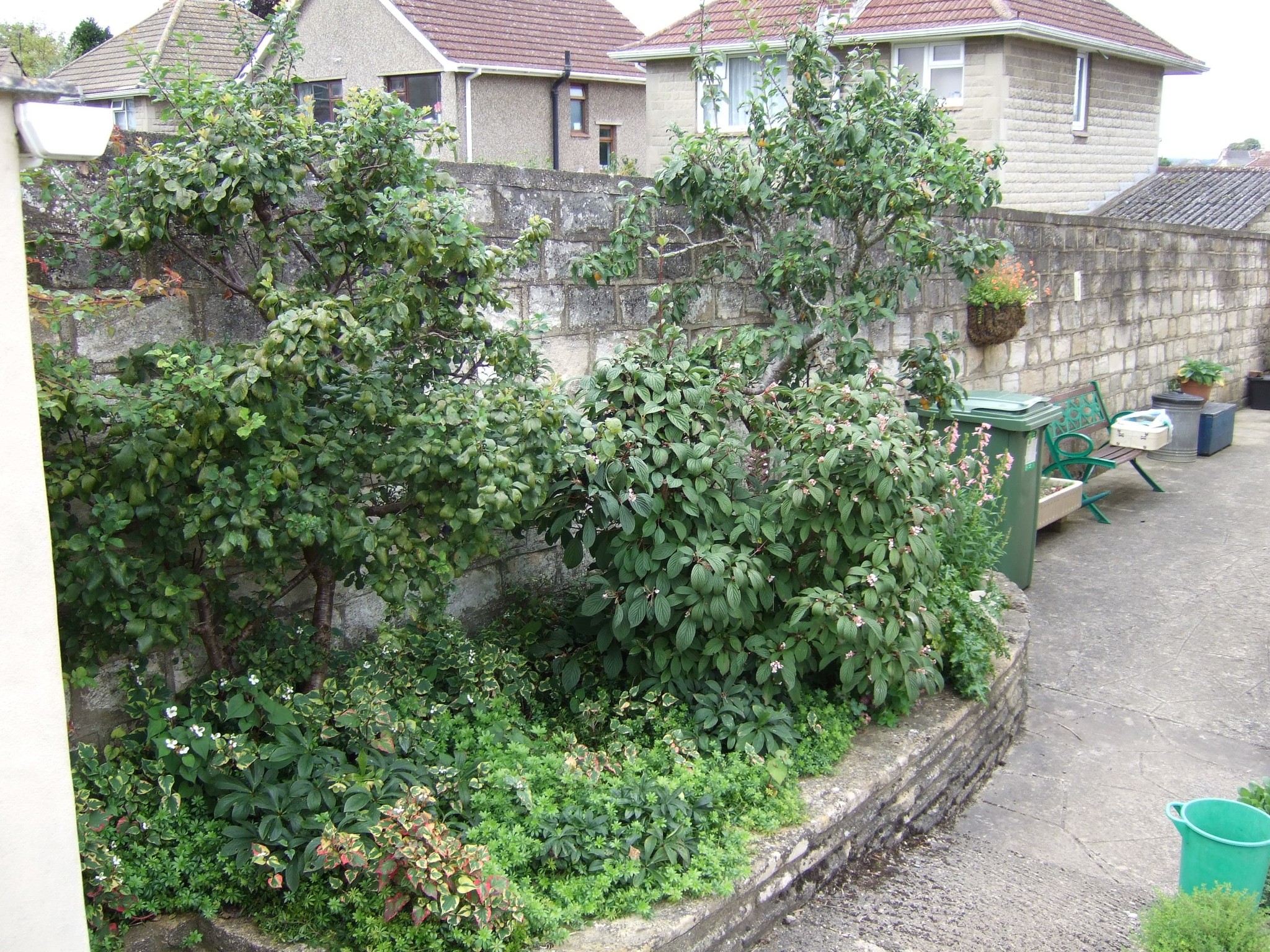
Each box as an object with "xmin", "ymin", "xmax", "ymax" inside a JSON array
[
  {"xmin": 1109, "ymin": 412, "xmax": 1174, "ymax": 451},
  {"xmin": 1246, "ymin": 374, "xmax": 1270, "ymax": 410},
  {"xmin": 1198, "ymin": 402, "xmax": 1238, "ymax": 456}
]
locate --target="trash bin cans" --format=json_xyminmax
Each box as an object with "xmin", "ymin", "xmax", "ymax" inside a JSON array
[
  {"xmin": 906, "ymin": 386, "xmax": 1066, "ymax": 592},
  {"xmin": 1148, "ymin": 391, "xmax": 1205, "ymax": 463}
]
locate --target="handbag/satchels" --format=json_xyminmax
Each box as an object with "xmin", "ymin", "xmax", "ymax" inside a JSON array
[{"xmin": 1116, "ymin": 409, "xmax": 1171, "ymax": 428}]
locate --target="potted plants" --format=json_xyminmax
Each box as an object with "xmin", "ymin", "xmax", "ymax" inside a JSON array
[
  {"xmin": 1036, "ymin": 475, "xmax": 1084, "ymax": 530},
  {"xmin": 1176, "ymin": 355, "xmax": 1234, "ymax": 407}
]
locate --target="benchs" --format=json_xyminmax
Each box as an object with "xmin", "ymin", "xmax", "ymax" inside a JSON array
[{"xmin": 1039, "ymin": 381, "xmax": 1165, "ymax": 525}]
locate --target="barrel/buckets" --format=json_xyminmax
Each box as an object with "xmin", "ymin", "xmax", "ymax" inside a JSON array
[
  {"xmin": 1146, "ymin": 388, "xmax": 1205, "ymax": 463},
  {"xmin": 1165, "ymin": 798, "xmax": 1270, "ymax": 915}
]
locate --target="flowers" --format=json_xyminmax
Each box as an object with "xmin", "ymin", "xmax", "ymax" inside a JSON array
[{"xmin": 967, "ymin": 252, "xmax": 1052, "ymax": 348}]
